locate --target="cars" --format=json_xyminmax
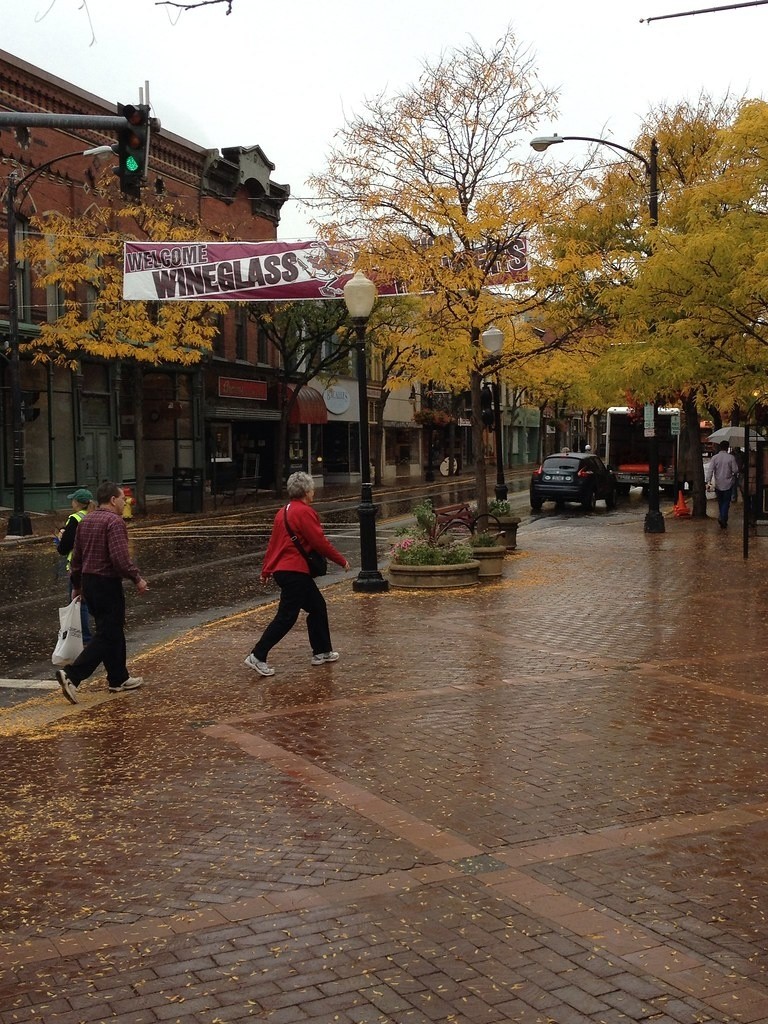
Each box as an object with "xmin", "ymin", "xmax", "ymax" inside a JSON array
[{"xmin": 529, "ymin": 449, "xmax": 620, "ymax": 514}]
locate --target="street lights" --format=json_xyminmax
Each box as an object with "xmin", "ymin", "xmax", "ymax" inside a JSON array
[
  {"xmin": 529, "ymin": 132, "xmax": 668, "ymax": 536},
  {"xmin": 480, "ymin": 325, "xmax": 509, "ymax": 501},
  {"xmin": 344, "ymin": 267, "xmax": 390, "ymax": 593}
]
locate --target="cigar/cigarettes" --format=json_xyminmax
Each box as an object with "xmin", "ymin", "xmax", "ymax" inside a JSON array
[{"xmin": 145, "ymin": 589, "xmax": 149, "ymax": 591}]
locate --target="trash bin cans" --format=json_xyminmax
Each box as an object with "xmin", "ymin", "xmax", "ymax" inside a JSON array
[{"xmin": 173, "ymin": 467, "xmax": 203, "ymax": 513}]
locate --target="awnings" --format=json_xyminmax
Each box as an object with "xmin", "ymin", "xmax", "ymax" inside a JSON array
[{"xmin": 277, "ymin": 383, "xmax": 327, "ymax": 422}]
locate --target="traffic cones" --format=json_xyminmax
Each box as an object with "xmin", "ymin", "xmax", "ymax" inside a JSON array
[{"xmin": 676, "ymin": 490, "xmax": 691, "ymax": 516}]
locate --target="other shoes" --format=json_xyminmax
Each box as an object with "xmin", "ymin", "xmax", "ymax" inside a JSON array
[{"xmin": 718, "ymin": 518, "xmax": 728, "ymax": 530}]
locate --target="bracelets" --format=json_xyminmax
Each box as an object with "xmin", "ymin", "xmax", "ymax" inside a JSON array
[{"xmin": 707, "ymin": 484, "xmax": 710, "ymax": 486}]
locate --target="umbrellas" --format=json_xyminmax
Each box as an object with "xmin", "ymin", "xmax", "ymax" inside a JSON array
[{"xmin": 705, "ymin": 424, "xmax": 765, "ymax": 447}]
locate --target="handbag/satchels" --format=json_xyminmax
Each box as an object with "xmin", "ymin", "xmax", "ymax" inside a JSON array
[
  {"xmin": 51, "ymin": 594, "xmax": 85, "ymax": 667},
  {"xmin": 307, "ymin": 550, "xmax": 329, "ymax": 579}
]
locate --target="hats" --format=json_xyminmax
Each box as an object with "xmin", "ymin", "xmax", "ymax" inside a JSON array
[{"xmin": 66, "ymin": 489, "xmax": 94, "ymax": 503}]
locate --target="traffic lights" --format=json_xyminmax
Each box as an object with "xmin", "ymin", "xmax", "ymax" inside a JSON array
[
  {"xmin": 120, "ymin": 103, "xmax": 150, "ymax": 177},
  {"xmin": 23, "ymin": 390, "xmax": 41, "ymax": 423}
]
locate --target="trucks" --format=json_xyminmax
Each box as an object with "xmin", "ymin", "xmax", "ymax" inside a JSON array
[{"xmin": 601, "ymin": 405, "xmax": 695, "ymax": 498}]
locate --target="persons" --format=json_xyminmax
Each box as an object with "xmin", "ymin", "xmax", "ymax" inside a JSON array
[
  {"xmin": 572, "ymin": 437, "xmax": 587, "ymax": 453},
  {"xmin": 243, "ymin": 472, "xmax": 350, "ymax": 676},
  {"xmin": 706, "ymin": 440, "xmax": 756, "ymax": 527},
  {"xmin": 56, "ymin": 482, "xmax": 146, "ymax": 705},
  {"xmin": 53, "ymin": 489, "xmax": 95, "ymax": 649}
]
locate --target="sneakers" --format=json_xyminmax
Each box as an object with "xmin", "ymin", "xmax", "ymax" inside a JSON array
[
  {"xmin": 109, "ymin": 675, "xmax": 142, "ymax": 691},
  {"xmin": 244, "ymin": 653, "xmax": 275, "ymax": 676},
  {"xmin": 311, "ymin": 651, "xmax": 339, "ymax": 665},
  {"xmin": 55, "ymin": 670, "xmax": 79, "ymax": 704}
]
